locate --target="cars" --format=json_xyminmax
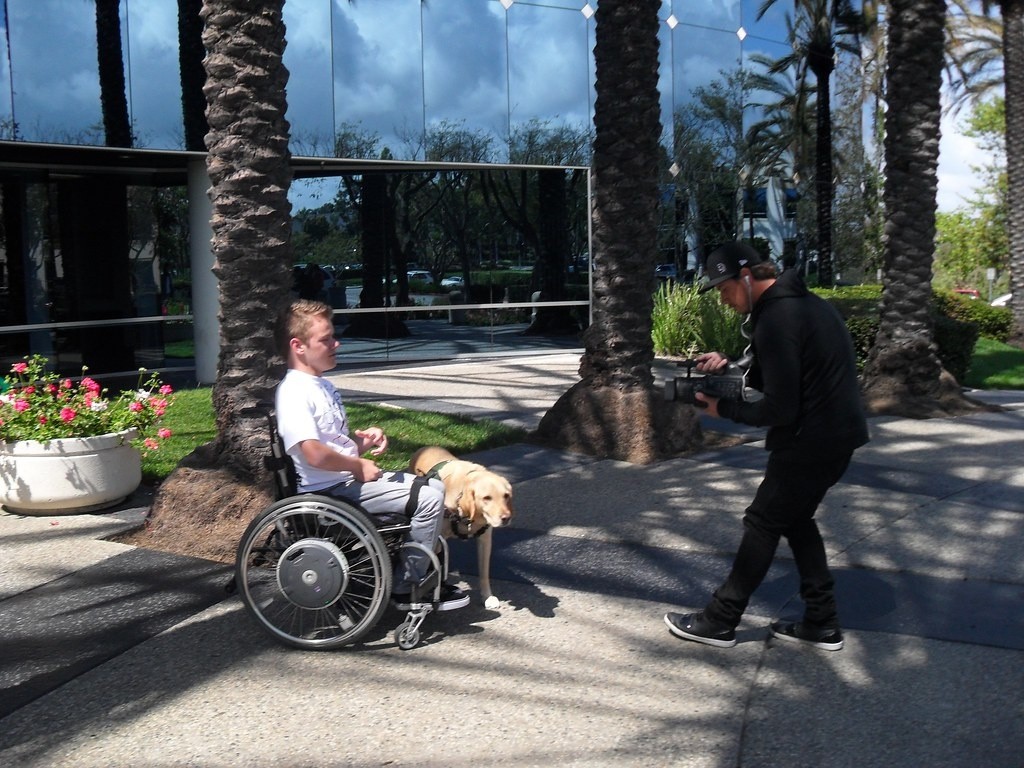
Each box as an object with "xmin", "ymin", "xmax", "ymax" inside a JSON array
[
  {"xmin": 654, "ymin": 264, "xmax": 676, "ymax": 276},
  {"xmin": 392, "ymin": 271, "xmax": 434, "ymax": 285},
  {"xmin": 989, "ymin": 293, "xmax": 1012, "ymax": 308},
  {"xmin": 951, "ymin": 289, "xmax": 981, "ymax": 300},
  {"xmin": 441, "ymin": 277, "xmax": 464, "ymax": 288}
]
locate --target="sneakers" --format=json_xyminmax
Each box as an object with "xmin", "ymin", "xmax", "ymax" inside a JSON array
[
  {"xmin": 392, "ymin": 581, "xmax": 470, "ymax": 611},
  {"xmin": 664, "ymin": 611, "xmax": 736, "ymax": 647},
  {"xmin": 769, "ymin": 620, "xmax": 843, "ymax": 650}
]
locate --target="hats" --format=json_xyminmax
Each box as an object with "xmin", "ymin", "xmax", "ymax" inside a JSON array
[{"xmin": 698, "ymin": 243, "xmax": 761, "ymax": 294}]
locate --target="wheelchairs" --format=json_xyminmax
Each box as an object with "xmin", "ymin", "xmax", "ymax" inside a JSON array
[{"xmin": 225, "ymin": 401, "xmax": 470, "ymax": 652}]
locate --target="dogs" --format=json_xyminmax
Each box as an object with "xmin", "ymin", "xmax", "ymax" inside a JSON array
[{"xmin": 408, "ymin": 446, "xmax": 514, "ymax": 609}]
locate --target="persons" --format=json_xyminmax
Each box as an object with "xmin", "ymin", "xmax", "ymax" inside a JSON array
[
  {"xmin": 293, "ymin": 263, "xmax": 322, "ymax": 296},
  {"xmin": 664, "ymin": 241, "xmax": 872, "ymax": 651},
  {"xmin": 275, "ymin": 299, "xmax": 470, "ymax": 611}
]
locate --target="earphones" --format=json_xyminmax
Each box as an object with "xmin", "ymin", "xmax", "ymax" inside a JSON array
[{"xmin": 744, "ymin": 276, "xmax": 751, "ymax": 286}]
